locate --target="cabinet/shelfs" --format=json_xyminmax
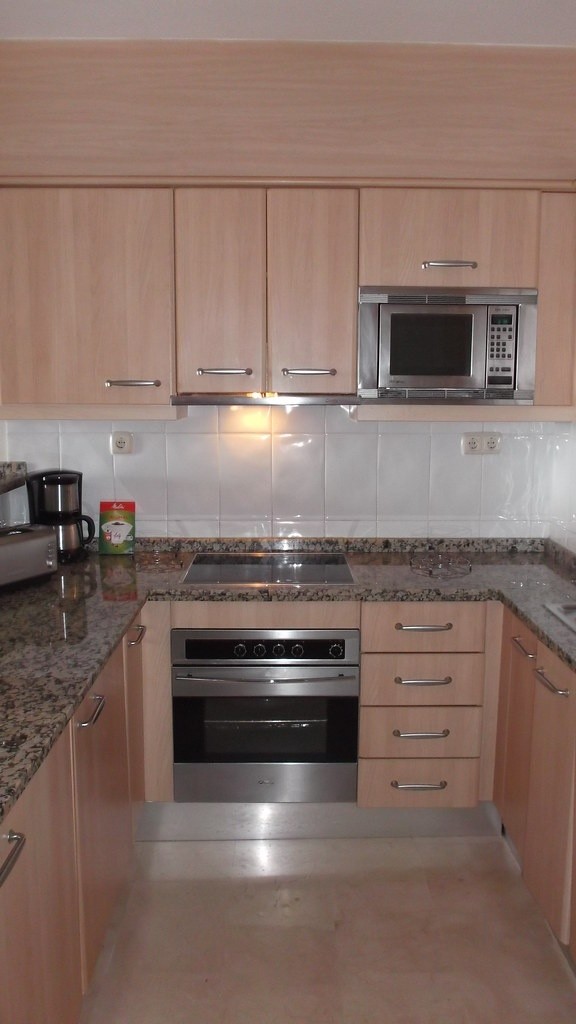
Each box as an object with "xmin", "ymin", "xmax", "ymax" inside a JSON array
[
  {"xmin": 358, "ymin": 188, "xmax": 540, "ymax": 290},
  {"xmin": 493, "ymin": 605, "xmax": 576, "ymax": 945},
  {"xmin": 122, "ymin": 611, "xmax": 145, "ymax": 842},
  {"xmin": 69, "ymin": 638, "xmax": 134, "ymax": 998},
  {"xmin": 0, "ymin": 720, "xmax": 84, "ymax": 1024},
  {"xmin": 0, "ymin": 186, "xmax": 187, "ymax": 421},
  {"xmin": 534, "ymin": 191, "xmax": 576, "ymax": 406},
  {"xmin": 172, "ymin": 187, "xmax": 359, "ymax": 396},
  {"xmin": 357, "ymin": 600, "xmax": 487, "ymax": 808}
]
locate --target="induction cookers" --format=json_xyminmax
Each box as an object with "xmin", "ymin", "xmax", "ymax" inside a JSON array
[{"xmin": 181, "ymin": 552, "xmax": 356, "ymax": 586}]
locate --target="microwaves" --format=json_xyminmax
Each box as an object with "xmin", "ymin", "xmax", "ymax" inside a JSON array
[{"xmin": 356, "ymin": 284, "xmax": 540, "ymax": 405}]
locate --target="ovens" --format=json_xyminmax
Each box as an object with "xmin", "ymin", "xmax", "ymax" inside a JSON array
[{"xmin": 173, "ymin": 629, "xmax": 360, "ymax": 803}]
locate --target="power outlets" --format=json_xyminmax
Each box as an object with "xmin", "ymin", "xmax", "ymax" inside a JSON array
[
  {"xmin": 110, "ymin": 430, "xmax": 134, "ymax": 454},
  {"xmin": 482, "ymin": 431, "xmax": 502, "ymax": 454},
  {"xmin": 461, "ymin": 432, "xmax": 483, "ymax": 455}
]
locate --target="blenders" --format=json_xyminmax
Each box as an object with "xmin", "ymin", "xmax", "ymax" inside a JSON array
[{"xmin": 25, "ymin": 468, "xmax": 95, "ymax": 564}]
